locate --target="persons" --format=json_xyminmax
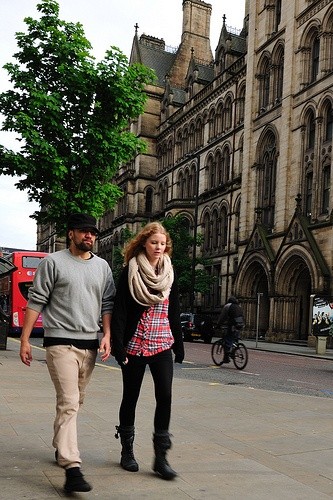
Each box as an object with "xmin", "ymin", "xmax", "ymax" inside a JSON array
[
  {"xmin": 110, "ymin": 221, "xmax": 185, "ymax": 478},
  {"xmin": 218, "ymin": 296, "xmax": 246, "ymax": 363},
  {"xmin": 20, "ymin": 213, "xmax": 117, "ymax": 493}
]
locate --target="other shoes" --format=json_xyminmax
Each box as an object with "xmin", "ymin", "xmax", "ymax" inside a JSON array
[
  {"xmin": 64, "ymin": 472, "xmax": 92, "ymax": 492},
  {"xmin": 222, "ymin": 358, "xmax": 230, "ymax": 363}
]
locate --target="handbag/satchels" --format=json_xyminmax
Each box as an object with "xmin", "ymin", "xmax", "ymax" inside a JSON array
[{"xmin": 233, "ymin": 316, "xmax": 245, "ymax": 329}]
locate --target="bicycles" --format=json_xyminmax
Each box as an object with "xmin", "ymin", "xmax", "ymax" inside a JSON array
[{"xmin": 210, "ymin": 327, "xmax": 249, "ymax": 370}]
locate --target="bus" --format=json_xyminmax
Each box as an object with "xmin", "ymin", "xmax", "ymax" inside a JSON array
[{"xmin": 0, "ymin": 251, "xmax": 52, "ymax": 338}]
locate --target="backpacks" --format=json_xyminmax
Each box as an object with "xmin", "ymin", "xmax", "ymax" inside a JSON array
[{"xmin": 225, "ymin": 300, "xmax": 243, "ymax": 317}]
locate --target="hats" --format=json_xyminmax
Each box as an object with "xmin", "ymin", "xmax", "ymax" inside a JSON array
[{"xmin": 67, "ymin": 213, "xmax": 101, "ymax": 236}]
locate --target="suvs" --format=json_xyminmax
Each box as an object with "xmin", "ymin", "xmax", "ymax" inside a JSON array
[{"xmin": 178, "ymin": 312, "xmax": 215, "ymax": 344}]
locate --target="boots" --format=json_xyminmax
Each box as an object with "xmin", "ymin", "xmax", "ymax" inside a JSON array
[
  {"xmin": 113, "ymin": 425, "xmax": 139, "ymax": 472},
  {"xmin": 152, "ymin": 433, "xmax": 179, "ymax": 479}
]
